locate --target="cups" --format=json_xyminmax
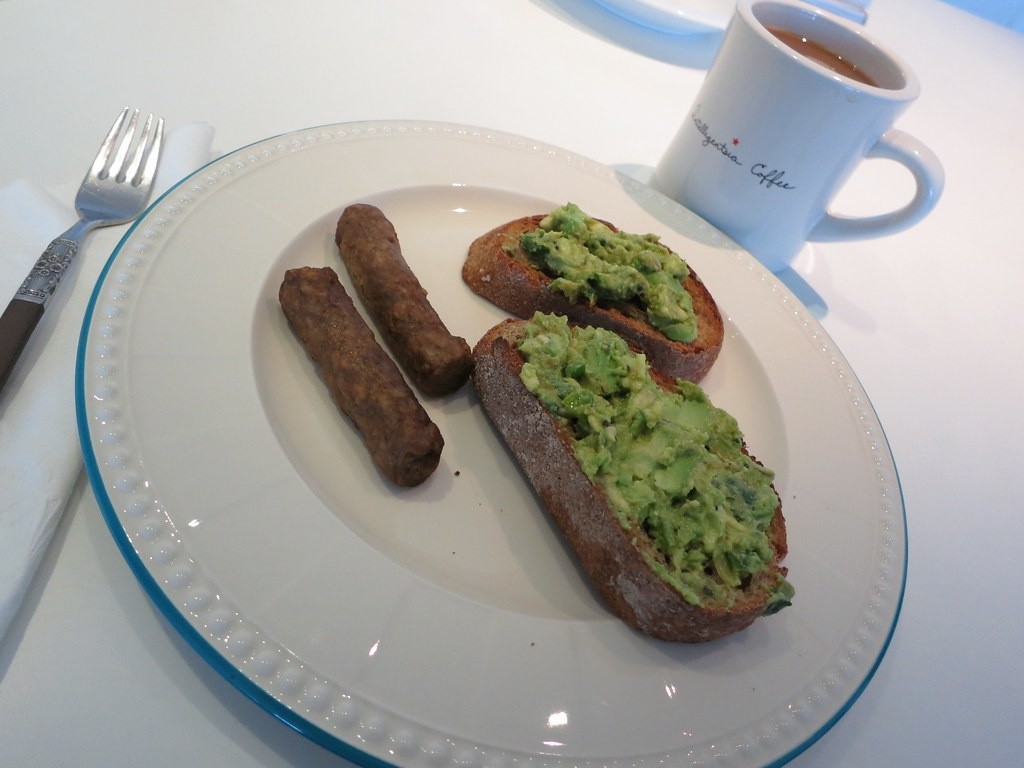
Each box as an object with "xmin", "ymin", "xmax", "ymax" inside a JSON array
[{"xmin": 652, "ymin": 0, "xmax": 947, "ymax": 272}]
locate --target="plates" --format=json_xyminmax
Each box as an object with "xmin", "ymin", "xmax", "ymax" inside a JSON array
[
  {"xmin": 74, "ymin": 117, "xmax": 910, "ymax": 768},
  {"xmin": 593, "ymin": 0, "xmax": 737, "ymax": 35}
]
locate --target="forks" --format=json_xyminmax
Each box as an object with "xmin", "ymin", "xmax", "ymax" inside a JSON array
[{"xmin": 0, "ymin": 105, "xmax": 163, "ymax": 391}]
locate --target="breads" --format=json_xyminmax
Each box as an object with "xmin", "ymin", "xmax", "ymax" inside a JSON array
[
  {"xmin": 472, "ymin": 318, "xmax": 787, "ymax": 641},
  {"xmin": 463, "ymin": 216, "xmax": 723, "ymax": 386}
]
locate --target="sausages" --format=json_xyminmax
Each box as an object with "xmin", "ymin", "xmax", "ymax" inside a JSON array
[
  {"xmin": 277, "ymin": 266, "xmax": 444, "ymax": 487},
  {"xmin": 333, "ymin": 204, "xmax": 474, "ymax": 394}
]
